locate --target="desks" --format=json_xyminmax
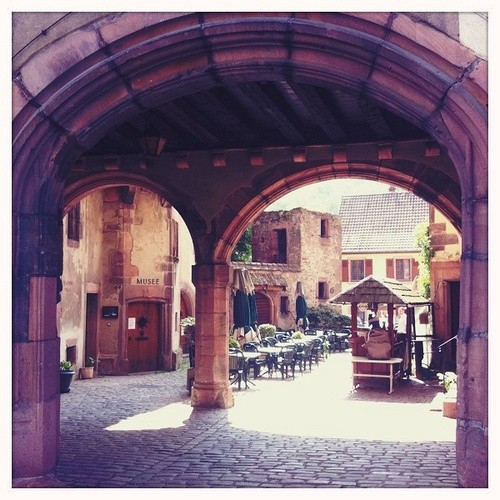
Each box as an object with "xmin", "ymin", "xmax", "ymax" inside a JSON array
[{"xmin": 346, "ymin": 355, "xmax": 403, "ymax": 394}]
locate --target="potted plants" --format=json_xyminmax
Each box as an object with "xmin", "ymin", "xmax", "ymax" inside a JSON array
[
  {"xmin": 59, "ymin": 359, "xmax": 76, "ymax": 394},
  {"xmin": 79, "ymin": 357, "xmax": 94, "ymax": 379}
]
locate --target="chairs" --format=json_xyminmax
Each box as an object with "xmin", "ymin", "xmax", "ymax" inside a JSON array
[{"xmin": 185, "ymin": 322, "xmax": 353, "ymax": 392}]
